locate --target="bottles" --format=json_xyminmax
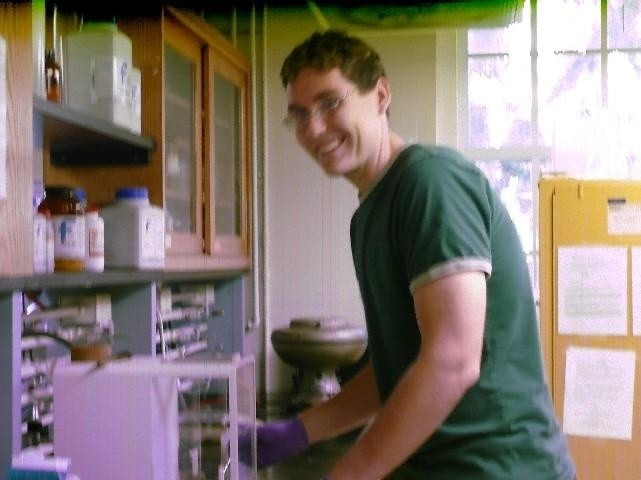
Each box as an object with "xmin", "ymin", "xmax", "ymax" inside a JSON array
[
  {"xmin": 45, "ymin": 48, "xmax": 62, "ymax": 103},
  {"xmin": 39, "ymin": 185, "xmax": 106, "ymax": 274}
]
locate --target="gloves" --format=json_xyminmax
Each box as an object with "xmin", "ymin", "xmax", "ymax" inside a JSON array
[{"xmin": 228, "ymin": 418, "xmax": 308, "ymax": 470}]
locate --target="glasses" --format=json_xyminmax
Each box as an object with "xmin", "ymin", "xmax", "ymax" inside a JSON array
[{"xmin": 280, "ymin": 88, "xmax": 357, "ymax": 131}]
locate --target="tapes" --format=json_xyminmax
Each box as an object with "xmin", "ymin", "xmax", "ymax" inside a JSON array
[{"xmin": 70, "ymin": 344, "xmax": 111, "ymax": 360}]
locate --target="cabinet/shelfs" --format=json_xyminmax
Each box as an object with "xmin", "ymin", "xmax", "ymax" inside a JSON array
[
  {"xmin": 43, "ymin": 1, "xmax": 161, "ymax": 272},
  {"xmin": 164, "ymin": 16, "xmax": 249, "ymax": 261}
]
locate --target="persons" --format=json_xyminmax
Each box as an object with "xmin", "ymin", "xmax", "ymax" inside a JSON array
[{"xmin": 229, "ymin": 29, "xmax": 579, "ymax": 479}]
